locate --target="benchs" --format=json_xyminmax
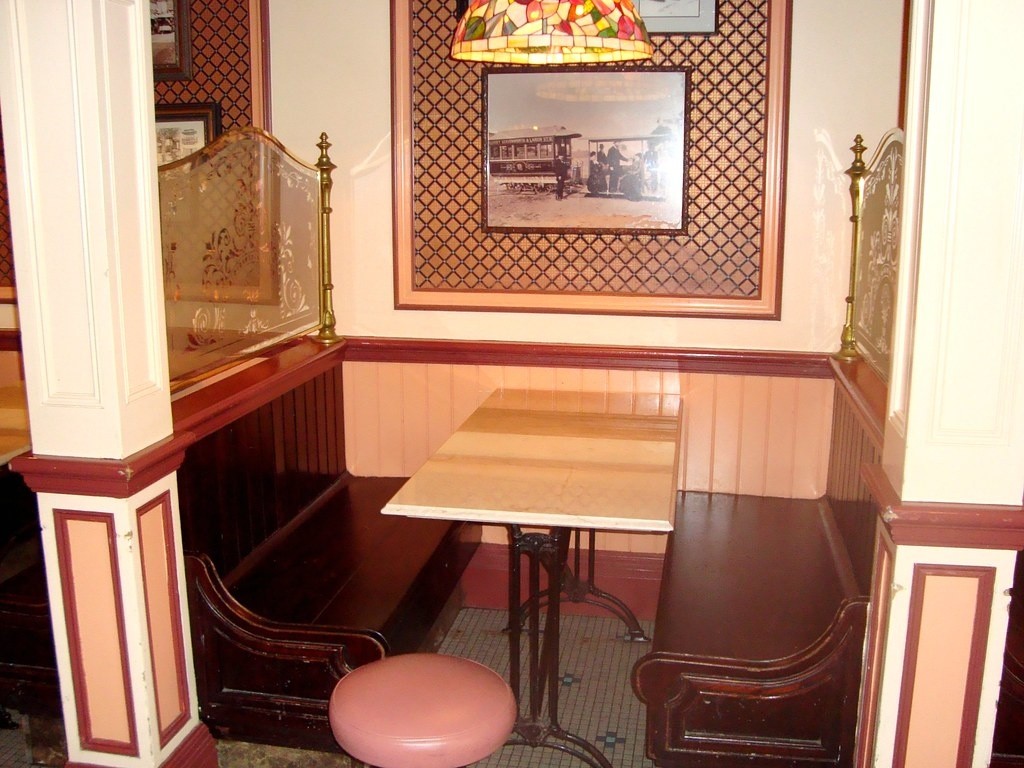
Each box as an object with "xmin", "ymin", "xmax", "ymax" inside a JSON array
[
  {"xmin": 628, "ymin": 356, "xmax": 882, "ymax": 768},
  {"xmin": 176, "ymin": 339, "xmax": 482, "ymax": 754}
]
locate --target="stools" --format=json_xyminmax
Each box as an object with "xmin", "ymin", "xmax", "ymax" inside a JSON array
[{"xmin": 329, "ymin": 652, "xmax": 518, "ymax": 767}]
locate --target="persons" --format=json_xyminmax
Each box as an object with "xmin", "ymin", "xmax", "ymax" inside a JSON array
[{"xmin": 555, "ymin": 140, "xmax": 658, "ymax": 202}]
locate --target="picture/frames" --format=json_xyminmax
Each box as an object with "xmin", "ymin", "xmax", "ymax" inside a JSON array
[
  {"xmin": 634, "ymin": 1, "xmax": 719, "ymax": 36},
  {"xmin": 150, "ymin": 0, "xmax": 193, "ymax": 82},
  {"xmin": 481, "ymin": 66, "xmax": 691, "ymax": 235},
  {"xmin": 155, "ymin": 103, "xmax": 222, "ymax": 168}
]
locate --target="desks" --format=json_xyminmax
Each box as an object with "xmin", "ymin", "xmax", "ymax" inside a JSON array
[{"xmin": 380, "ymin": 388, "xmax": 686, "ymax": 768}]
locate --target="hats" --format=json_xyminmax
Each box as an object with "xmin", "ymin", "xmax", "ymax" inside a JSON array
[{"xmin": 556, "ymin": 151, "xmax": 566, "ymax": 158}]
